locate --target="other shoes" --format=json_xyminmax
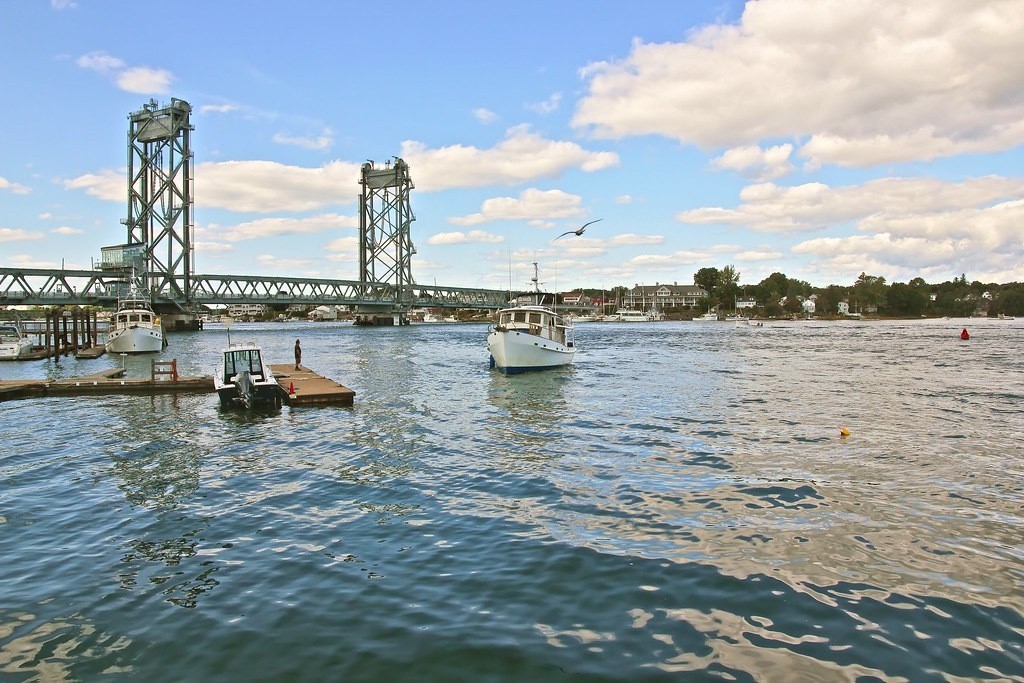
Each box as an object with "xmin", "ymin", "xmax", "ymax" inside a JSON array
[{"xmin": 295, "ymin": 368, "xmax": 301, "ymax": 371}]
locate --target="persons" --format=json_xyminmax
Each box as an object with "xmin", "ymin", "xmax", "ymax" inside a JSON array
[{"xmin": 295, "ymin": 339, "xmax": 302, "ymax": 371}]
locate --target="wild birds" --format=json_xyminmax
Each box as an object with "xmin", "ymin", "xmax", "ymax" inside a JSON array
[{"xmin": 554, "ymin": 218, "xmax": 603, "ymax": 242}]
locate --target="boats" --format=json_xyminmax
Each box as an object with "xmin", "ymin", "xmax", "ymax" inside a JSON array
[
  {"xmin": 212, "ymin": 326, "xmax": 282, "ymax": 411},
  {"xmin": 487, "ymin": 261, "xmax": 577, "ymax": 376},
  {"xmin": 692, "ymin": 310, "xmax": 750, "ymax": 323},
  {"xmin": 1, "ymin": 327, "xmax": 35, "ymax": 360},
  {"xmin": 199, "ymin": 312, "xmax": 324, "ymax": 324},
  {"xmin": 403, "ymin": 308, "xmax": 458, "ymax": 326},
  {"xmin": 107, "ymin": 263, "xmax": 162, "ymax": 354},
  {"xmin": 565, "ymin": 310, "xmax": 654, "ymax": 324}
]
